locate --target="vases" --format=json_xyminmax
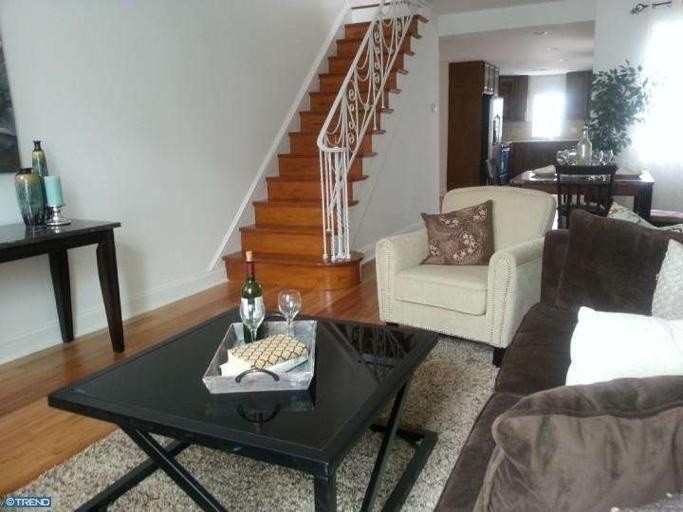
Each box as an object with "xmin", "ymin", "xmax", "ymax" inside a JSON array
[{"xmin": 32, "ymin": 141, "xmax": 52, "ymax": 222}]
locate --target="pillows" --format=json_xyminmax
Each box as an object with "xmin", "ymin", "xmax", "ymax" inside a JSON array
[
  {"xmin": 606, "ymin": 201, "xmax": 682, "ymax": 318},
  {"xmin": 553, "ymin": 208, "xmax": 670, "ymax": 316},
  {"xmin": 419, "ymin": 200, "xmax": 495, "ymax": 265},
  {"xmin": 565, "ymin": 306, "xmax": 682, "ymax": 385},
  {"xmin": 474, "ymin": 376, "xmax": 683, "ymax": 512}
]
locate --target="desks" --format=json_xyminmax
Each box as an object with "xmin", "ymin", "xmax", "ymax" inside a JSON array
[
  {"xmin": 0, "ymin": 219, "xmax": 124, "ymax": 353},
  {"xmin": 509, "ymin": 170, "xmax": 655, "ymax": 221}
]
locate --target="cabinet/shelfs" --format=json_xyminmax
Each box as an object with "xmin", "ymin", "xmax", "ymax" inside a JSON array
[
  {"xmin": 566, "ymin": 71, "xmax": 591, "ymax": 121},
  {"xmin": 499, "ymin": 75, "xmax": 528, "ymax": 121},
  {"xmin": 447, "ymin": 60, "xmax": 504, "ymax": 192}
]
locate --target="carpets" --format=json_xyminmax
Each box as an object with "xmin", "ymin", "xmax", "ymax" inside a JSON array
[{"xmin": 1, "ymin": 337, "xmax": 501, "ymax": 512}]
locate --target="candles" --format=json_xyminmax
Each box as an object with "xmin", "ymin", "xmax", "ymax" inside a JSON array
[{"xmin": 44, "ymin": 175, "xmax": 63, "ymax": 205}]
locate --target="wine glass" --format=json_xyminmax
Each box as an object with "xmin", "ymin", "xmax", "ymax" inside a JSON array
[
  {"xmin": 557, "ymin": 139, "xmax": 615, "ymax": 170},
  {"xmin": 277, "ymin": 289, "xmax": 302, "ymax": 350},
  {"xmin": 240, "ymin": 302, "xmax": 266, "ymax": 351}
]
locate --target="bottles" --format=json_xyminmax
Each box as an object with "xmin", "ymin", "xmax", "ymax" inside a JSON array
[
  {"xmin": 575, "ymin": 126, "xmax": 593, "ymax": 167},
  {"xmin": 240, "ymin": 251, "xmax": 268, "ymax": 353},
  {"xmin": 27, "ymin": 141, "xmax": 55, "ymax": 222}
]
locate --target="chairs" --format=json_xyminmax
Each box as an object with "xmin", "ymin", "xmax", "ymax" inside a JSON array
[
  {"xmin": 555, "ymin": 164, "xmax": 616, "ymax": 229},
  {"xmin": 650, "ymin": 208, "xmax": 683, "ymax": 227},
  {"xmin": 375, "ymin": 186, "xmax": 556, "ymax": 367}
]
